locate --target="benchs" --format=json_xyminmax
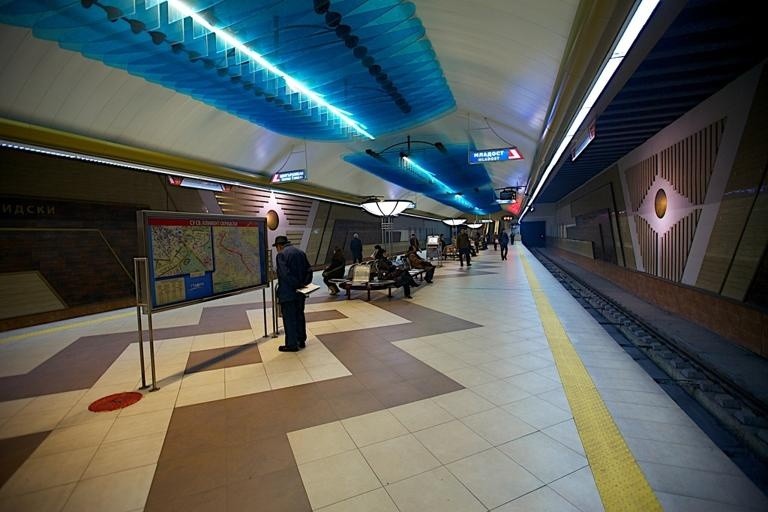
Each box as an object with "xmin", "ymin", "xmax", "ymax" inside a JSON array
[{"xmin": 328, "ymin": 254, "xmax": 426, "ymax": 302}]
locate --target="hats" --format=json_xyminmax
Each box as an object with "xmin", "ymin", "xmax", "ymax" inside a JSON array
[{"xmin": 272, "ymin": 236, "xmax": 290, "ymax": 246}]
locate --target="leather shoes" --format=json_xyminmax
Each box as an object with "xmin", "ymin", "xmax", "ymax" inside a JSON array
[
  {"xmin": 415, "ymin": 283, "xmax": 421, "ymax": 287},
  {"xmin": 407, "ymin": 295, "xmax": 412, "ymax": 298},
  {"xmin": 279, "ymin": 341, "xmax": 305, "ymax": 352}
]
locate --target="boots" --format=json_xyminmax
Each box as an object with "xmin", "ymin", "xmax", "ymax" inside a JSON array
[{"xmin": 328, "ymin": 284, "xmax": 340, "ymax": 295}]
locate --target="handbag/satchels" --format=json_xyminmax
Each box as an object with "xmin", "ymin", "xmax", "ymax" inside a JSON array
[{"xmin": 322, "ymin": 268, "xmax": 334, "ymax": 277}]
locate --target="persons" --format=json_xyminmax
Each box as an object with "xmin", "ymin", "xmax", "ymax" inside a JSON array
[
  {"xmin": 370, "ymin": 227, "xmax": 515, "ymax": 299},
  {"xmin": 350, "ymin": 233, "xmax": 363, "ymax": 263},
  {"xmin": 272, "ymin": 236, "xmax": 313, "ymax": 352},
  {"xmin": 323, "ymin": 246, "xmax": 345, "ymax": 295}
]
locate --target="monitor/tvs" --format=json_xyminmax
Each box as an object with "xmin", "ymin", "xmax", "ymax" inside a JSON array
[
  {"xmin": 500, "ymin": 189, "xmax": 517, "ymax": 200},
  {"xmin": 428, "ymin": 236, "xmax": 438, "ymax": 244}
]
